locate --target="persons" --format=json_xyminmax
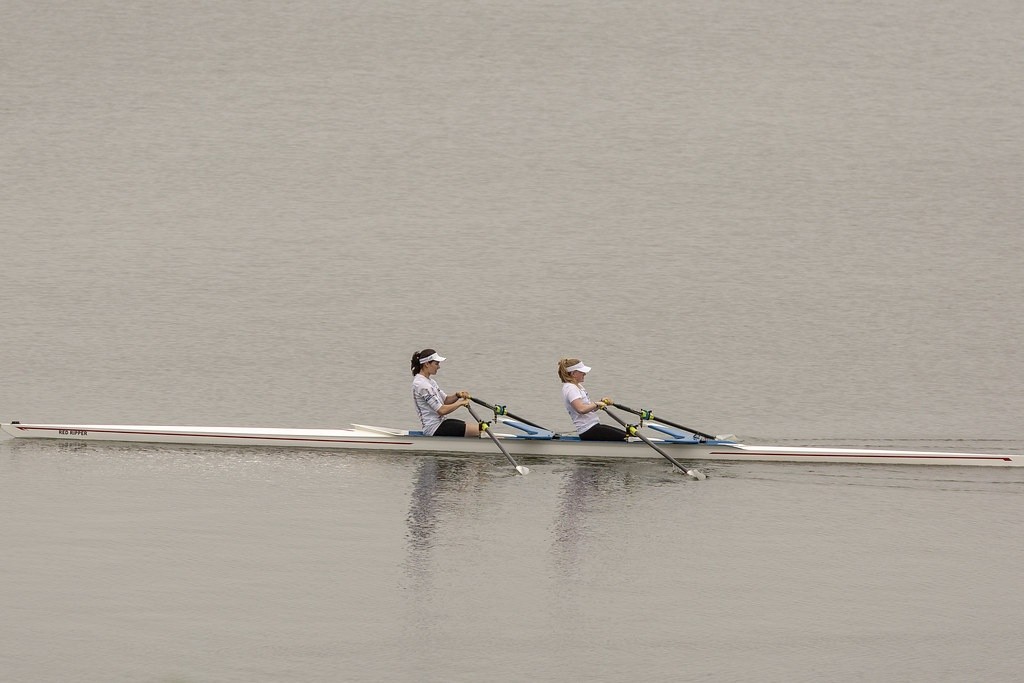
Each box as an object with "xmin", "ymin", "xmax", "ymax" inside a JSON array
[
  {"xmin": 559, "ymin": 360, "xmax": 637, "ymax": 441},
  {"xmin": 411, "ymin": 349, "xmax": 480, "ymax": 437}
]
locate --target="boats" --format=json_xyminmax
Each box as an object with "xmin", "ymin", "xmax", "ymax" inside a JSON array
[{"xmin": 0, "ymin": 419, "xmax": 1023, "ymax": 474}]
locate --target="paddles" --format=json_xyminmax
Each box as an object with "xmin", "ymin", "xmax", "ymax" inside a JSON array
[
  {"xmin": 604, "ymin": 400, "xmax": 738, "ymax": 441},
  {"xmin": 603, "ymin": 405, "xmax": 707, "ymax": 481},
  {"xmin": 470, "ymin": 395, "xmax": 561, "ymax": 435},
  {"xmin": 467, "ymin": 405, "xmax": 529, "ymax": 475}
]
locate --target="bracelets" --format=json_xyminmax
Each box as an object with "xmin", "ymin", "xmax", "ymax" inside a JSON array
[
  {"xmin": 456, "ymin": 393, "xmax": 460, "ymax": 398},
  {"xmin": 594, "ymin": 402, "xmax": 597, "ymax": 410},
  {"xmin": 600, "ymin": 397, "xmax": 603, "ymax": 402}
]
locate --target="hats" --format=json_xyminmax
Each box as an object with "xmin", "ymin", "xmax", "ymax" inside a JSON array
[
  {"xmin": 419, "ymin": 352, "xmax": 446, "ymax": 364},
  {"xmin": 566, "ymin": 361, "xmax": 591, "ymax": 373}
]
